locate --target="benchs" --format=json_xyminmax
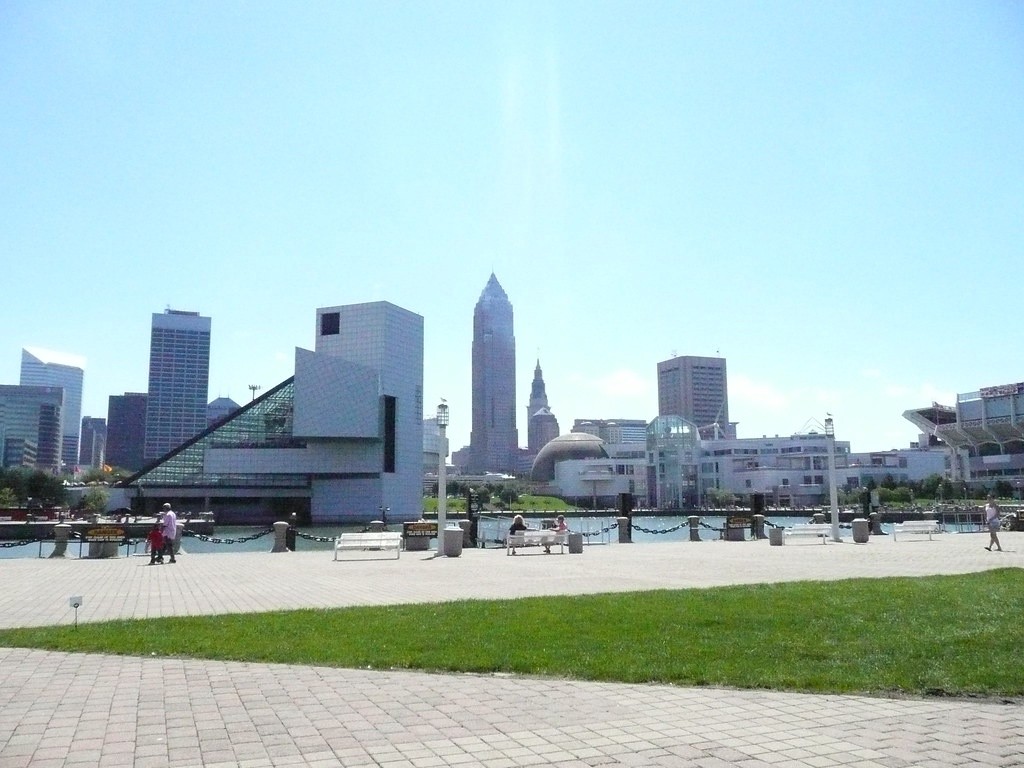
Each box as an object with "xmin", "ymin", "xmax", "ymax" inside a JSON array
[
  {"xmin": 892, "ymin": 519, "xmax": 940, "ymax": 541},
  {"xmin": 781, "ymin": 523, "xmax": 833, "ymax": 545},
  {"xmin": 506, "ymin": 529, "xmax": 567, "ymax": 555},
  {"xmin": 335, "ymin": 532, "xmax": 403, "ymax": 560}
]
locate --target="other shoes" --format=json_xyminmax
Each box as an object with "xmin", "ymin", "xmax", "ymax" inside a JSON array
[
  {"xmin": 994, "ymin": 548, "xmax": 1002, "ymax": 551},
  {"xmin": 984, "ymin": 547, "xmax": 993, "ymax": 552},
  {"xmin": 543, "ymin": 549, "xmax": 550, "ymax": 554},
  {"xmin": 512, "ymin": 551, "xmax": 516, "ymax": 555},
  {"xmin": 148, "ymin": 561, "xmax": 156, "ymax": 565}
]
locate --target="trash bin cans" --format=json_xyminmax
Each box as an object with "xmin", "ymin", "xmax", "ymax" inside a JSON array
[
  {"xmin": 851, "ymin": 517, "xmax": 869, "ymax": 543},
  {"xmin": 442, "ymin": 525, "xmax": 464, "ymax": 557}
]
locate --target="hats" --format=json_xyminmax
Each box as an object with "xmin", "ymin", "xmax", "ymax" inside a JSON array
[{"xmin": 162, "ymin": 503, "xmax": 171, "ymax": 509}]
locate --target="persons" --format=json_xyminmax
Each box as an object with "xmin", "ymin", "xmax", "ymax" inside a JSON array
[
  {"xmin": 543, "ymin": 515, "xmax": 567, "ymax": 554},
  {"xmin": 984, "ymin": 494, "xmax": 1003, "ymax": 551},
  {"xmin": 509, "ymin": 514, "xmax": 527, "ymax": 556},
  {"xmin": 146, "ymin": 524, "xmax": 164, "ymax": 565},
  {"xmin": 155, "ymin": 503, "xmax": 176, "ymax": 564}
]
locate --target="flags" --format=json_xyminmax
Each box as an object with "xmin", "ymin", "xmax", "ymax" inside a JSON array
[
  {"xmin": 74, "ymin": 466, "xmax": 80, "ymax": 472},
  {"xmin": 103, "ymin": 465, "xmax": 112, "ymax": 473}
]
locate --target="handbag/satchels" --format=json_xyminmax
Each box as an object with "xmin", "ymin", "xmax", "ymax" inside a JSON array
[{"xmin": 988, "ymin": 516, "xmax": 1002, "ymax": 533}]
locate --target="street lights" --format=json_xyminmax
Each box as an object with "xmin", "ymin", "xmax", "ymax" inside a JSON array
[
  {"xmin": 436, "ymin": 403, "xmax": 449, "ymax": 556},
  {"xmin": 825, "ymin": 417, "xmax": 841, "ymax": 542}
]
